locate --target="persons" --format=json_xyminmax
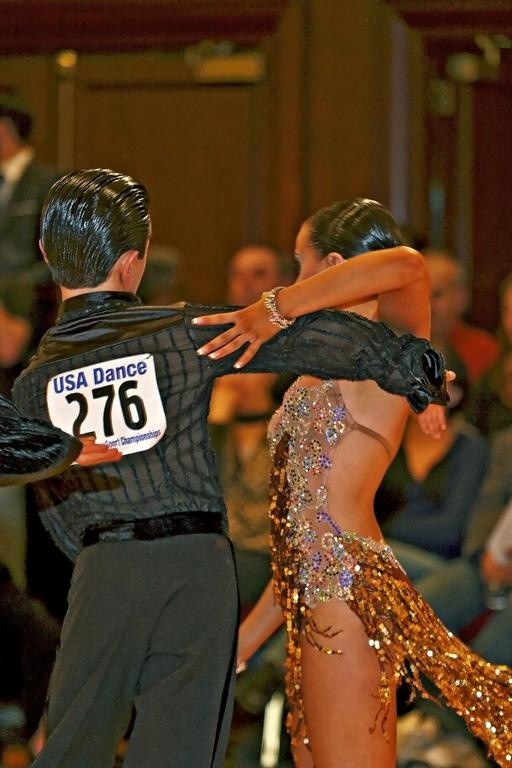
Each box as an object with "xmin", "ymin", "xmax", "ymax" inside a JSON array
[
  {"xmin": 0, "ymin": 240, "xmax": 510, "ymax": 768},
  {"xmin": 1, "ymin": 104, "xmax": 68, "ymax": 401},
  {"xmin": 191, "ymin": 197, "xmax": 511, "ymax": 768},
  {"xmin": 0, "ymin": 168, "xmax": 455, "ymax": 768}
]
